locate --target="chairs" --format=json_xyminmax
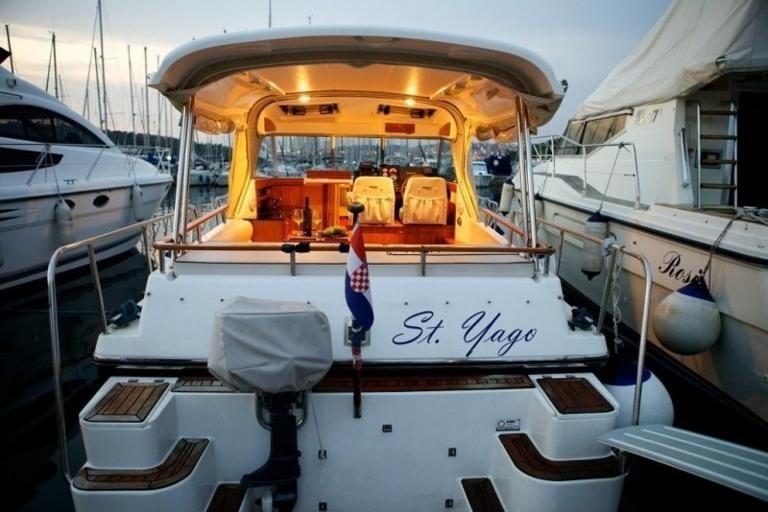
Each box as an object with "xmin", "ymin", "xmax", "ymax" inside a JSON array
[{"xmin": 343, "ymin": 174, "xmax": 450, "ymax": 228}]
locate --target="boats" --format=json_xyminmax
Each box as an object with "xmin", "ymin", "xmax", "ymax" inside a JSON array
[
  {"xmin": 1, "ymin": 47, "xmax": 172, "ymax": 291},
  {"xmin": 492, "ymin": 0, "xmax": 767, "ymax": 419}
]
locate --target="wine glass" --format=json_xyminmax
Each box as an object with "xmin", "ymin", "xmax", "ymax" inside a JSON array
[{"xmin": 291, "ymin": 208, "xmax": 304, "ymax": 239}]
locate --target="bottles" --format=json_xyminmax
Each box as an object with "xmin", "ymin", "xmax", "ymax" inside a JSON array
[{"xmin": 302, "ymin": 196, "xmax": 313, "ymax": 238}]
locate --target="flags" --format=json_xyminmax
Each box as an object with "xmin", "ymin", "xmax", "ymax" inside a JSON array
[{"xmin": 345, "ymin": 214, "xmax": 374, "ymax": 331}]
